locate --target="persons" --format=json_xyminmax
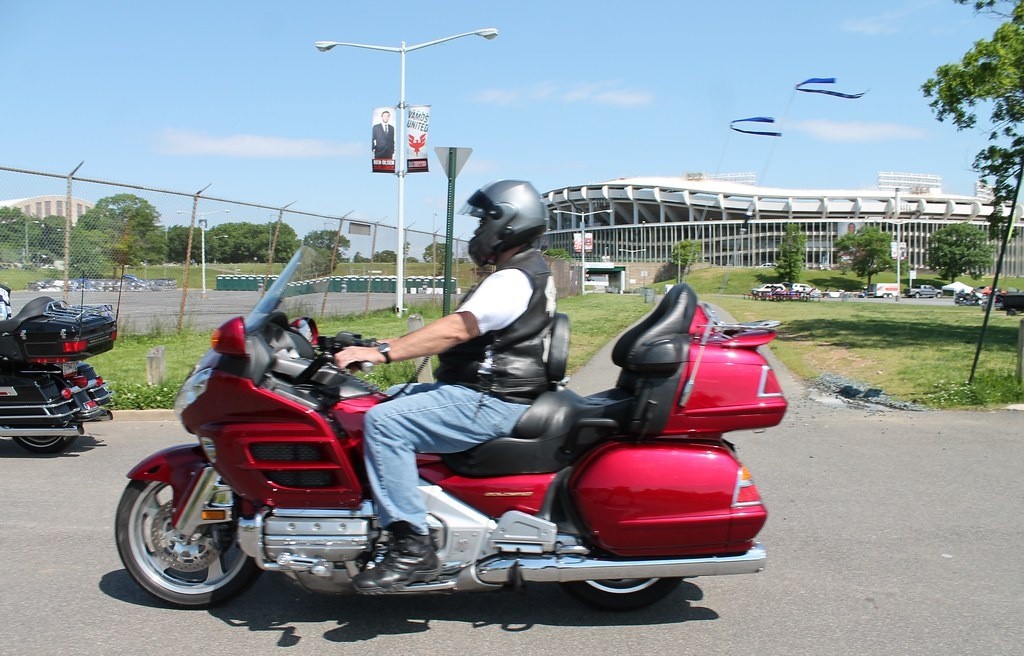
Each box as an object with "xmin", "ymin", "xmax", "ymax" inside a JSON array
[
  {"xmin": 847, "ymin": 222, "xmax": 856, "ymax": 234},
  {"xmin": 372, "ymin": 111, "xmax": 394, "ymax": 158},
  {"xmin": 334, "ymin": 180, "xmax": 559, "ymax": 595}
]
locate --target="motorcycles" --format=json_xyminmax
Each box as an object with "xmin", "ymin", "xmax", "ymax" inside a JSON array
[
  {"xmin": 0, "ymin": 295, "xmax": 117, "ymax": 452},
  {"xmin": 112, "ymin": 244, "xmax": 789, "ymax": 614}
]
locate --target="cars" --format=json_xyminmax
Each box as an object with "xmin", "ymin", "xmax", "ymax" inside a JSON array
[
  {"xmin": 752, "ymin": 282, "xmax": 820, "ymax": 300},
  {"xmin": 957, "ymin": 286, "xmax": 1024, "ymax": 315},
  {"xmin": 27, "ymin": 274, "xmax": 177, "ymax": 294},
  {"xmin": 758, "ymin": 262, "xmax": 775, "ymax": 267}
]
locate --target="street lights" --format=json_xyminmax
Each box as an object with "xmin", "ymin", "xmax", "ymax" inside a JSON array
[
  {"xmin": 552, "ymin": 209, "xmax": 613, "ymax": 295},
  {"xmin": 314, "ymin": 26, "xmax": 498, "ymax": 316},
  {"xmin": 619, "ymin": 248, "xmax": 647, "ymax": 264},
  {"xmin": 177, "ymin": 208, "xmax": 231, "ymax": 298}
]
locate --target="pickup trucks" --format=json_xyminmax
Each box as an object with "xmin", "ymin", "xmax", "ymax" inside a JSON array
[{"xmin": 904, "ymin": 285, "xmax": 944, "ymax": 298}]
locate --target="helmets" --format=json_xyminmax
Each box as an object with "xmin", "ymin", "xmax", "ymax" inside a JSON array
[{"xmin": 455, "ymin": 180, "xmax": 551, "ymax": 268}]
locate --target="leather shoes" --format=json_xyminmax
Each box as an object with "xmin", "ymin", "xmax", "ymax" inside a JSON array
[{"xmin": 351, "ymin": 526, "xmax": 441, "ymax": 594}]
